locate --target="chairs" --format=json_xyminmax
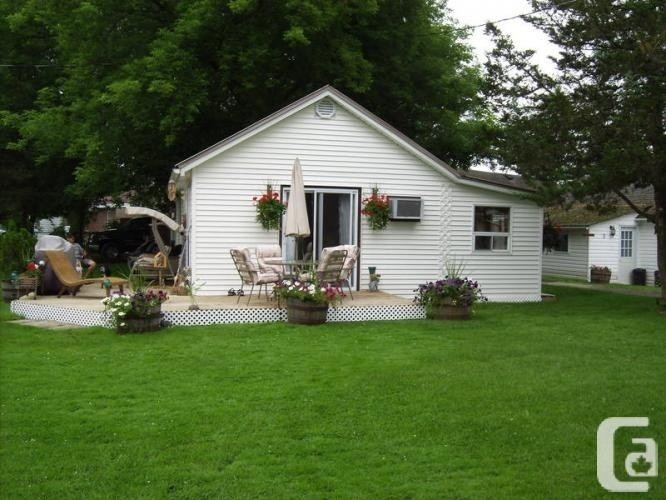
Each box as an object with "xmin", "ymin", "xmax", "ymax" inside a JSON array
[
  {"xmin": 254, "ymin": 245, "xmax": 286, "ymax": 301},
  {"xmin": 231, "ymin": 248, "xmax": 280, "ymax": 305},
  {"xmin": 44, "ymin": 251, "xmax": 129, "ymax": 300},
  {"xmin": 318, "ymin": 249, "xmax": 346, "ymax": 300},
  {"xmin": 326, "ymin": 245, "xmax": 359, "ymax": 300}
]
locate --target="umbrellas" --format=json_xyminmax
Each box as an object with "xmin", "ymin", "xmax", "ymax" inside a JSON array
[{"xmin": 284, "ymin": 155, "xmax": 309, "ymax": 279}]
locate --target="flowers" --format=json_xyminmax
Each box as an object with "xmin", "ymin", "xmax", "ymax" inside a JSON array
[
  {"xmin": 276, "ymin": 271, "xmax": 335, "ymax": 301},
  {"xmin": 360, "ymin": 186, "xmax": 391, "ymax": 230},
  {"xmin": 413, "ymin": 275, "xmax": 489, "ymax": 306},
  {"xmin": 254, "ymin": 186, "xmax": 290, "ymax": 228},
  {"xmin": 113, "ymin": 272, "xmax": 169, "ymax": 308}
]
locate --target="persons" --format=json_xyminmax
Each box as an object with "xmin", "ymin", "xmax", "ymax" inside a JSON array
[{"xmin": 65, "ymin": 233, "xmax": 95, "ymax": 279}]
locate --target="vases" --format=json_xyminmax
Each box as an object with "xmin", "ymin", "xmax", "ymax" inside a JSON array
[
  {"xmin": 114, "ymin": 304, "xmax": 161, "ymax": 332},
  {"xmin": 432, "ymin": 295, "xmax": 473, "ymax": 318},
  {"xmin": 286, "ymin": 296, "xmax": 329, "ymax": 323}
]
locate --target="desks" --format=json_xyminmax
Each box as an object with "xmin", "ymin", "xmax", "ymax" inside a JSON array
[{"xmin": 265, "ymin": 257, "xmax": 318, "ymax": 291}]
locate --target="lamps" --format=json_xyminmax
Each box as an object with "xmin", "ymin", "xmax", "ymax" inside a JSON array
[{"xmin": 609, "ymin": 226, "xmax": 616, "ymax": 236}]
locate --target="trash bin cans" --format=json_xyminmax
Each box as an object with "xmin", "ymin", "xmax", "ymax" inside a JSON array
[{"xmin": 632, "ymin": 268, "xmax": 646, "ymax": 286}]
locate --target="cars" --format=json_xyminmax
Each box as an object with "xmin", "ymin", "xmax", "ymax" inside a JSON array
[{"xmin": 87, "ymin": 216, "xmax": 170, "ymax": 260}]
